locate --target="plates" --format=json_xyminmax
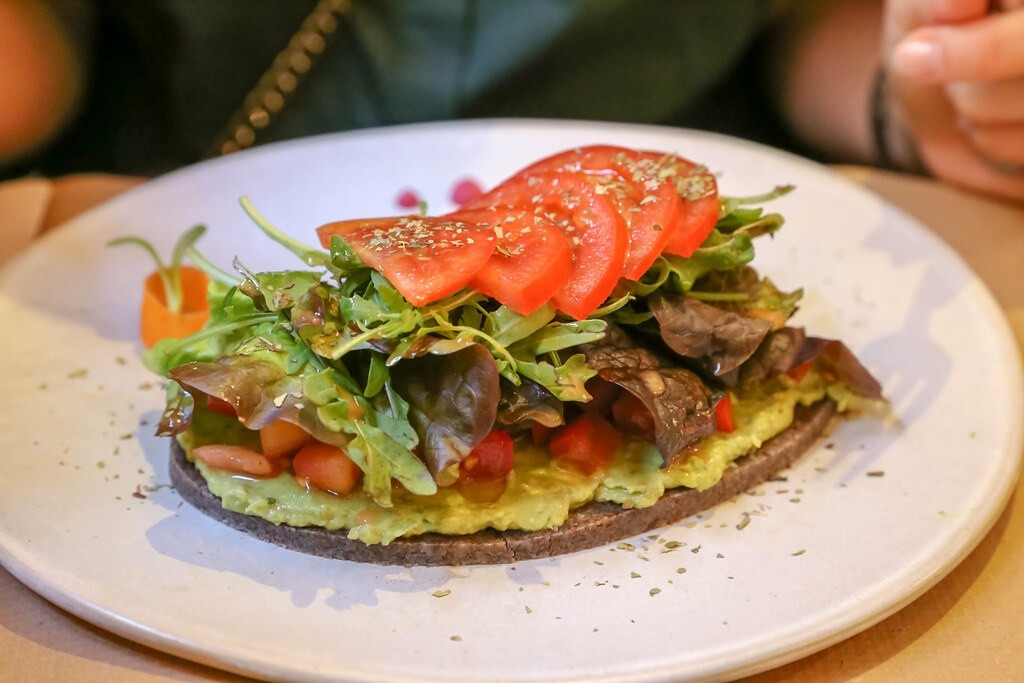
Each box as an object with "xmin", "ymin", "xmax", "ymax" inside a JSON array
[{"xmin": 0, "ymin": 117, "xmax": 1024, "ymax": 682}]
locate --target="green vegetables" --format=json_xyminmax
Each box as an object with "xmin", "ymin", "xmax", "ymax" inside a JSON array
[{"xmin": 104, "ymin": 184, "xmax": 887, "ymax": 511}]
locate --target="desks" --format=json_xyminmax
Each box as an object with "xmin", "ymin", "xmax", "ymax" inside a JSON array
[{"xmin": 0, "ymin": 163, "xmax": 1024, "ymax": 683}]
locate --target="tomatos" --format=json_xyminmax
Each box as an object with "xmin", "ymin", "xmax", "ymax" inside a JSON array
[{"xmin": 315, "ymin": 143, "xmax": 719, "ymax": 321}]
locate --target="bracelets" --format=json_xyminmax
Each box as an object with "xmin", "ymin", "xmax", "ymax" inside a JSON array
[{"xmin": 866, "ymin": 68, "xmax": 897, "ymax": 175}]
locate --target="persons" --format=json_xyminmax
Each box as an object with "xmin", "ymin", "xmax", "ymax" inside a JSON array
[{"xmin": 1, "ymin": 0, "xmax": 1019, "ymax": 207}]
